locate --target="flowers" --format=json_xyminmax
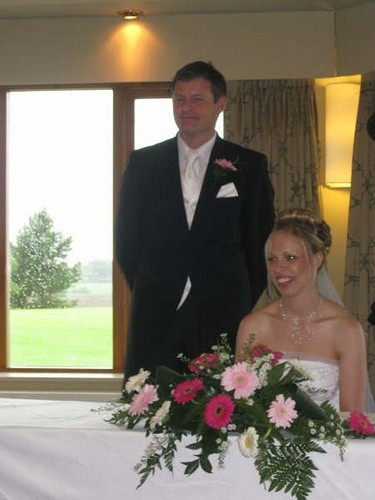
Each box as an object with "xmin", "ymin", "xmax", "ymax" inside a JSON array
[
  {"xmin": 90, "ymin": 331, "xmax": 375, "ymax": 500},
  {"xmin": 211, "ymin": 156, "xmax": 240, "ymax": 179}
]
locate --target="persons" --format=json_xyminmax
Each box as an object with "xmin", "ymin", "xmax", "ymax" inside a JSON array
[
  {"xmin": 235, "ymin": 209, "xmax": 375, "ymax": 412},
  {"xmin": 115, "ymin": 60, "xmax": 276, "ymax": 406}
]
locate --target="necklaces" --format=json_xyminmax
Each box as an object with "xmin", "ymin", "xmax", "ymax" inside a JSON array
[{"xmin": 279, "ymin": 294, "xmax": 325, "ymax": 345}]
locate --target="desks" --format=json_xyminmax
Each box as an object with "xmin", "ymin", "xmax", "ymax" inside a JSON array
[{"xmin": 0, "ymin": 398, "xmax": 375, "ymax": 500}]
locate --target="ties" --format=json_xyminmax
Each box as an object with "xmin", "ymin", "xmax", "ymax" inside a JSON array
[{"xmin": 175, "ymin": 151, "xmax": 201, "ymax": 311}]
